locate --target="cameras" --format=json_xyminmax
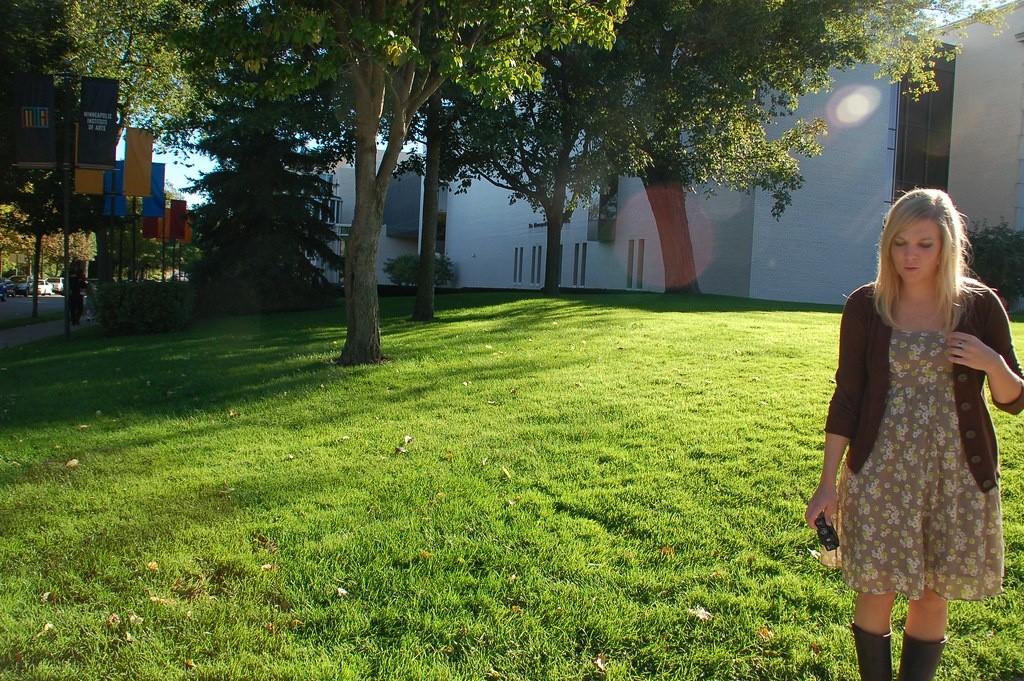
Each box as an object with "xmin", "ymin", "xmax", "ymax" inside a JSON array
[{"xmin": 815, "ymin": 517, "xmax": 840, "ymax": 551}]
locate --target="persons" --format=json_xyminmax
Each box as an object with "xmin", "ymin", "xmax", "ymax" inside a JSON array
[
  {"xmin": 62, "ymin": 257, "xmax": 87, "ymax": 326},
  {"xmin": 806, "ymin": 188, "xmax": 1024, "ymax": 681}
]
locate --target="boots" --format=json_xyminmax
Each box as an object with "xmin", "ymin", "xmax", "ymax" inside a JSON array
[
  {"xmin": 898, "ymin": 630, "xmax": 949, "ymax": 681},
  {"xmin": 71, "ymin": 312, "xmax": 76, "ymax": 325},
  {"xmin": 76, "ymin": 313, "xmax": 80, "ymax": 325},
  {"xmin": 852, "ymin": 623, "xmax": 892, "ymax": 681}
]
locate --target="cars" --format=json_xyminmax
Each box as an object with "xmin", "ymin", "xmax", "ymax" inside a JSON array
[
  {"xmin": 38, "ymin": 278, "xmax": 53, "ymax": 296},
  {"xmin": 0, "ymin": 277, "xmax": 17, "ymax": 302},
  {"xmin": 9, "ymin": 275, "xmax": 34, "ymax": 297}
]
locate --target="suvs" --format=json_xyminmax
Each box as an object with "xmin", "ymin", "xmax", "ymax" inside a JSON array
[{"xmin": 47, "ymin": 276, "xmax": 65, "ymax": 294}]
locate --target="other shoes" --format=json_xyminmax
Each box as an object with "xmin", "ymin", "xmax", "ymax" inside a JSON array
[
  {"xmin": 91, "ymin": 319, "xmax": 95, "ymax": 322},
  {"xmin": 86, "ymin": 319, "xmax": 90, "ymax": 322}
]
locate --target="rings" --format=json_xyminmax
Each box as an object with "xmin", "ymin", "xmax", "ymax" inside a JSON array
[{"xmin": 958, "ymin": 340, "xmax": 962, "ymax": 348}]
